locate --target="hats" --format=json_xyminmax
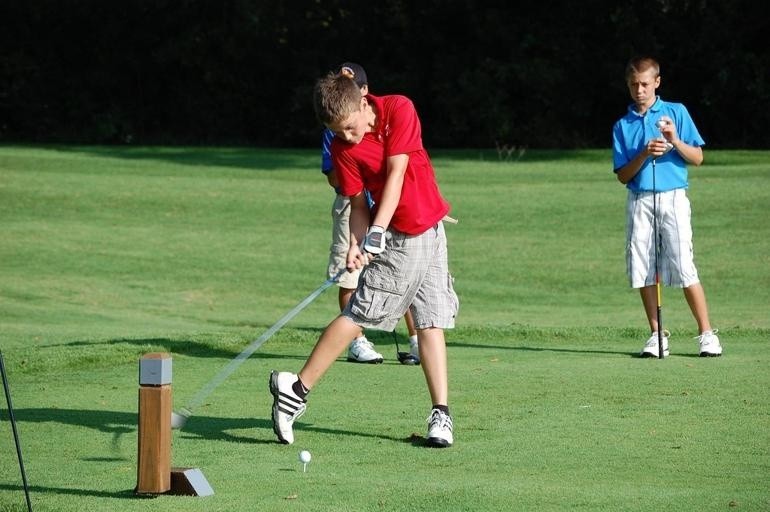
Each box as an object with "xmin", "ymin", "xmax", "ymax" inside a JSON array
[{"xmin": 327, "ymin": 58, "xmax": 371, "ymax": 90}]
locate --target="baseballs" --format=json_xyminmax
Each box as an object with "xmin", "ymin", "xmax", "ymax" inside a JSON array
[{"xmin": 655, "ymin": 118, "xmax": 667, "ymax": 128}]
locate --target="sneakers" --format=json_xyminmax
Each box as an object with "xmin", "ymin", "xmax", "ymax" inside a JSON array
[
  {"xmin": 405, "ymin": 334, "xmax": 445, "ymax": 359},
  {"xmin": 345, "ymin": 335, "xmax": 387, "ymax": 366},
  {"xmin": 696, "ymin": 329, "xmax": 725, "ymax": 358},
  {"xmin": 425, "ymin": 411, "xmax": 454, "ymax": 449},
  {"xmin": 265, "ymin": 368, "xmax": 306, "ymax": 446},
  {"xmin": 640, "ymin": 331, "xmax": 671, "ymax": 361}
]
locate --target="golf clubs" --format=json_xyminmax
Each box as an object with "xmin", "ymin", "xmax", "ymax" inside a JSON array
[
  {"xmin": 653, "ymin": 142, "xmax": 674, "ymax": 358},
  {"xmin": 393, "ymin": 329, "xmax": 420, "ymax": 365},
  {"xmin": 170, "ymin": 269, "xmax": 350, "ymax": 431}
]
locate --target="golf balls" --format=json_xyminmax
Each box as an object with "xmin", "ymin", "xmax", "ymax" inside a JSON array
[{"xmin": 299, "ymin": 450, "xmax": 312, "ymax": 463}]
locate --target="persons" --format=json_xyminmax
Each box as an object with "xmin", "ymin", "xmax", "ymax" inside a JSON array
[
  {"xmin": 613, "ymin": 57, "xmax": 724, "ymax": 357},
  {"xmin": 321, "ymin": 62, "xmax": 422, "ymax": 362},
  {"xmin": 269, "ymin": 75, "xmax": 460, "ymax": 447}
]
